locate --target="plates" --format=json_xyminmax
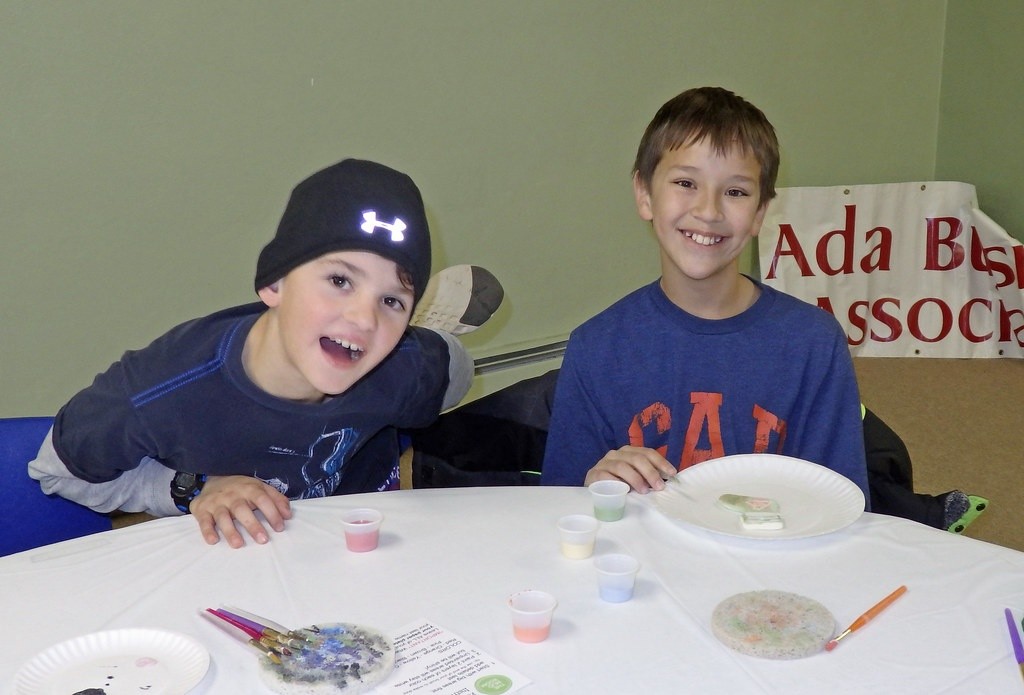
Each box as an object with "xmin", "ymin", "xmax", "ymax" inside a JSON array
[
  {"xmin": 656, "ymin": 453, "xmax": 866, "ymax": 541},
  {"xmin": 9, "ymin": 626, "xmax": 212, "ymax": 694}
]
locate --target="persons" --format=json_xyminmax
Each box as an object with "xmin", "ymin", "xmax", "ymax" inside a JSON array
[
  {"xmin": 538, "ymin": 86, "xmax": 872, "ymax": 513},
  {"xmin": 26, "ymin": 159, "xmax": 475, "ymax": 548}
]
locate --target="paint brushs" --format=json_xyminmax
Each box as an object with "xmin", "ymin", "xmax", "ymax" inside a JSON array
[
  {"xmin": 1005, "ymin": 608, "xmax": 1023, "ymax": 681},
  {"xmin": 827, "ymin": 585, "xmax": 907, "ymax": 652},
  {"xmin": 200, "ymin": 605, "xmax": 317, "ymax": 664}
]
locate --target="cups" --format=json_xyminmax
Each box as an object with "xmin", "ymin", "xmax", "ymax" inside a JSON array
[
  {"xmin": 506, "ymin": 590, "xmax": 557, "ymax": 642},
  {"xmin": 556, "ymin": 515, "xmax": 598, "ymax": 559},
  {"xmin": 589, "ymin": 480, "xmax": 630, "ymax": 522},
  {"xmin": 593, "ymin": 555, "xmax": 640, "ymax": 603},
  {"xmin": 341, "ymin": 508, "xmax": 383, "ymax": 551}
]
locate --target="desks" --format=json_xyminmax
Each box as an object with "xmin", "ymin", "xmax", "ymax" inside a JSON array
[{"xmin": 0, "ymin": 468, "xmax": 1024, "ymax": 695}]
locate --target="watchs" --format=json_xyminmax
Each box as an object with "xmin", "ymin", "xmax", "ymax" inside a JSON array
[{"xmin": 170, "ymin": 471, "xmax": 206, "ymax": 514}]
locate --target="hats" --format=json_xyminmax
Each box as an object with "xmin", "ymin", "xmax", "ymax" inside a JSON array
[{"xmin": 254, "ymin": 159, "xmax": 432, "ymax": 304}]
